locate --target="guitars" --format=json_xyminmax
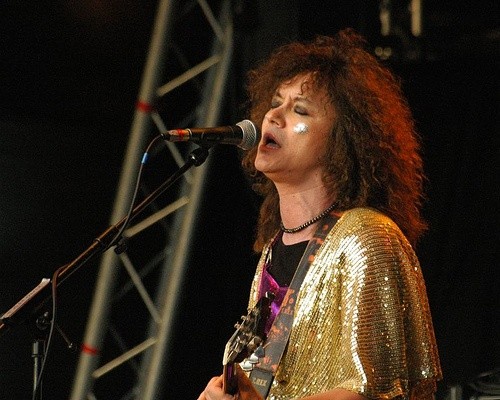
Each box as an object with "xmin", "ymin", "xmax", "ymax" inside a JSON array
[{"xmin": 222, "ymin": 293, "xmax": 274, "ymax": 396}]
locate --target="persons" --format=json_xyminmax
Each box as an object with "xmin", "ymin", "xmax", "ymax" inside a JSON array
[{"xmin": 196, "ymin": 27, "xmax": 443, "ymax": 400}]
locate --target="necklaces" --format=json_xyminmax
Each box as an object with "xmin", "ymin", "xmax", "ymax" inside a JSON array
[{"xmin": 279, "ymin": 197, "xmax": 338, "ymax": 233}]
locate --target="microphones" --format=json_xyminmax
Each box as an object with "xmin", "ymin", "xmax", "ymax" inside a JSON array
[{"xmin": 161, "ymin": 119, "xmax": 261, "ymax": 151}]
